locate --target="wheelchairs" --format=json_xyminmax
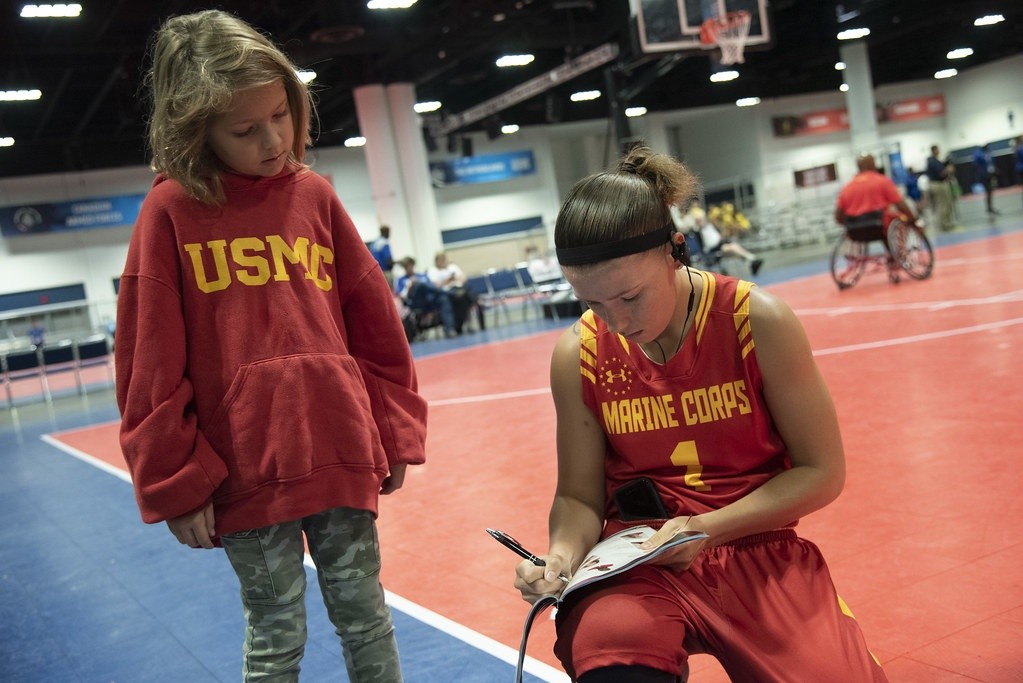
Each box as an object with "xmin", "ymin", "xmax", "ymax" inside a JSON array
[{"xmin": 830, "ymin": 208, "xmax": 935, "ymax": 287}]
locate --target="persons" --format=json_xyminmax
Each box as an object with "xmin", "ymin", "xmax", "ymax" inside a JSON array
[
  {"xmin": 117, "ymin": 11, "xmax": 429, "ymax": 683},
  {"xmin": 1016, "ymin": 136, "xmax": 1023, "ymax": 179},
  {"xmin": 514, "ymin": 145, "xmax": 889, "ymax": 683},
  {"xmin": 396, "ymin": 257, "xmax": 456, "ymax": 337},
  {"xmin": 833, "ymin": 153, "xmax": 920, "ymax": 283},
  {"xmin": 369, "ymin": 226, "xmax": 394, "ymax": 290},
  {"xmin": 427, "ymin": 253, "xmax": 475, "ymax": 335},
  {"xmin": 27, "ymin": 321, "xmax": 45, "ymax": 348},
  {"xmin": 676, "ymin": 198, "xmax": 763, "ymax": 277},
  {"xmin": 927, "ymin": 146, "xmax": 956, "ymax": 233},
  {"xmin": 973, "ymin": 144, "xmax": 998, "ymax": 216},
  {"xmin": 907, "ymin": 168, "xmax": 922, "ymax": 200}
]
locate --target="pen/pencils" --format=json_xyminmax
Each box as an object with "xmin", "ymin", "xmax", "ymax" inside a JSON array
[{"xmin": 485, "ymin": 527, "xmax": 570, "ymax": 584}]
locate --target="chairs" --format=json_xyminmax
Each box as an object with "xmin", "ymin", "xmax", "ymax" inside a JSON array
[{"xmin": 462, "ymin": 267, "xmax": 561, "ymax": 332}]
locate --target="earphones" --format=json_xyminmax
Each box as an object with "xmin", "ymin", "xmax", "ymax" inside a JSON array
[{"xmin": 670, "ymin": 241, "xmax": 680, "ymax": 261}]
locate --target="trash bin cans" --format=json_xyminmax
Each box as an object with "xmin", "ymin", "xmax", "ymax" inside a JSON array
[
  {"xmin": 948, "ymin": 145, "xmax": 991, "ymax": 192},
  {"xmin": 989, "ymin": 135, "xmax": 1023, "ymax": 186}
]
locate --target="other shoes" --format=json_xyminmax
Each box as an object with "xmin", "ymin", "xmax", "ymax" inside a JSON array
[
  {"xmin": 750, "ymin": 258, "xmax": 762, "ymax": 275},
  {"xmin": 986, "ymin": 208, "xmax": 997, "ymax": 214}
]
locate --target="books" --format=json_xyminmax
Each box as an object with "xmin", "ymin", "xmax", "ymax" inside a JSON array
[{"xmin": 515, "ymin": 524, "xmax": 709, "ymax": 683}]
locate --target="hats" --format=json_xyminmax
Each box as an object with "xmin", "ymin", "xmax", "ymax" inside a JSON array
[{"xmin": 400, "ymin": 257, "xmax": 414, "ymax": 265}]
